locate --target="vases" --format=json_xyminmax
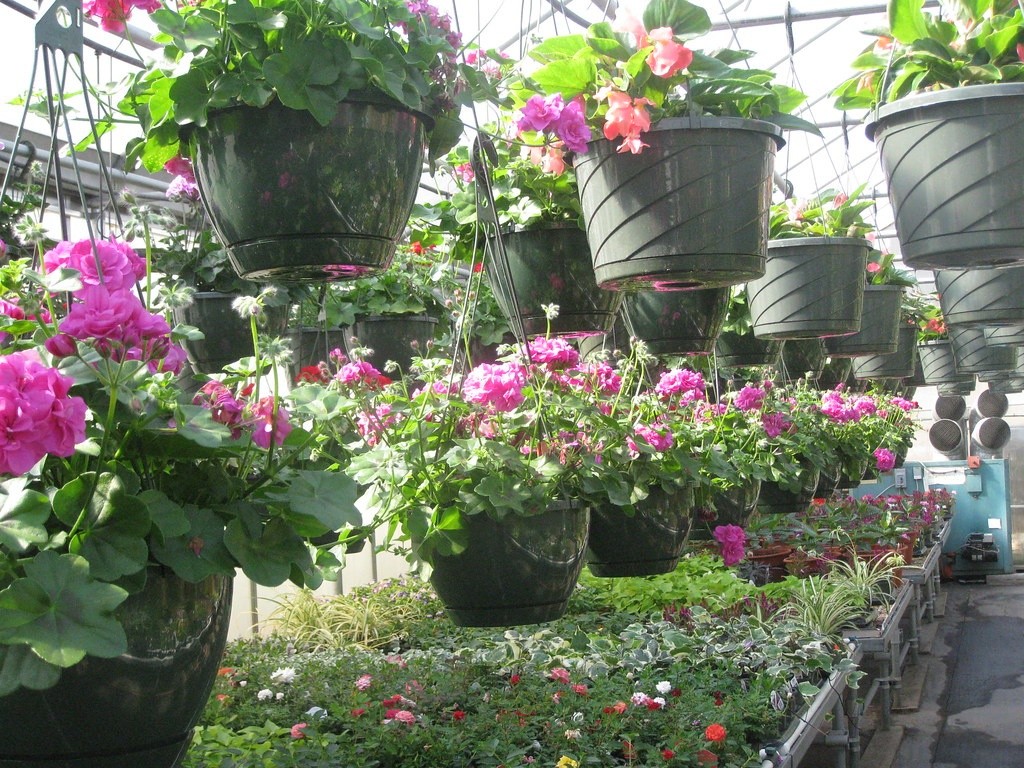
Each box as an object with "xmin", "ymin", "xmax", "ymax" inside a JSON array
[{"xmin": 0, "ymin": 77, "xmax": 1023, "ymax": 768}]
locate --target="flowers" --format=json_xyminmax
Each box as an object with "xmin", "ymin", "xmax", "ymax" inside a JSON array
[
  {"xmin": 0, "ymin": 0, "xmax": 953, "ymax": 768},
  {"xmin": 825, "ymin": 0, "xmax": 1024, "ymax": 121}
]
